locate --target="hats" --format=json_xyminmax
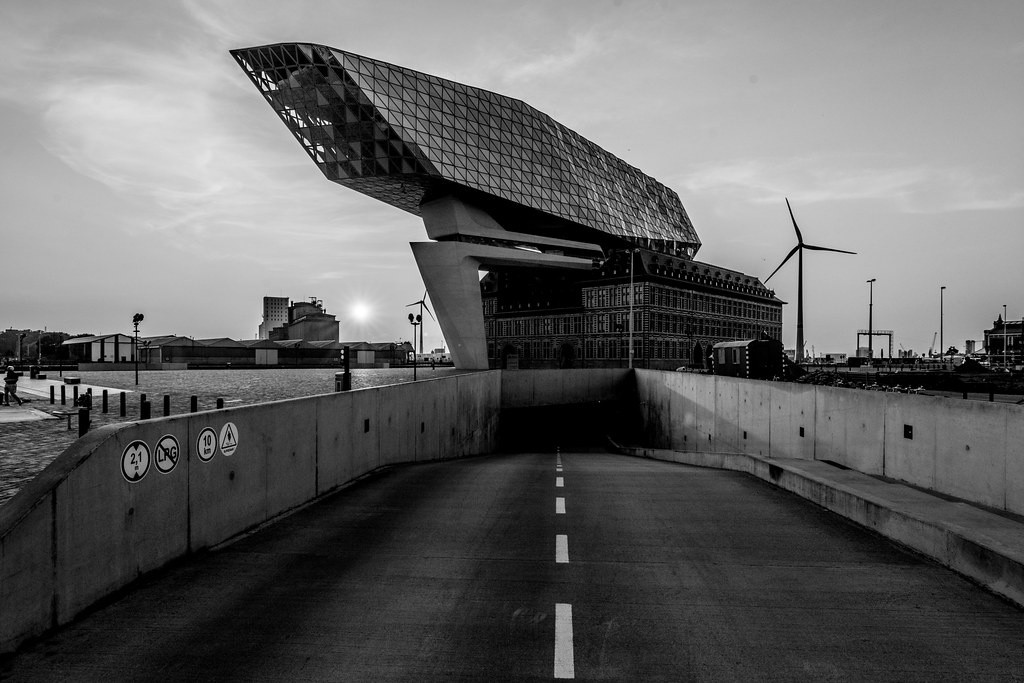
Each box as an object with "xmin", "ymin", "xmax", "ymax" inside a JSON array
[{"xmin": 7, "ymin": 366, "xmax": 14, "ymax": 370}]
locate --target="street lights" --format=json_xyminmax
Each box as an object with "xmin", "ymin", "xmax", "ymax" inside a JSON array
[
  {"xmin": 408, "ymin": 312, "xmax": 422, "ymax": 380},
  {"xmin": 867, "ymin": 278, "xmax": 877, "ymax": 360},
  {"xmin": 132, "ymin": 312, "xmax": 145, "ymax": 386},
  {"xmin": 1001, "ymin": 303, "xmax": 1007, "ymax": 369},
  {"xmin": 940, "ymin": 286, "xmax": 946, "ymax": 364}
]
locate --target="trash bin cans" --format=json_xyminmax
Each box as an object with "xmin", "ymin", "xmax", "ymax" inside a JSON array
[
  {"xmin": 335, "ymin": 372, "xmax": 351, "ymax": 392},
  {"xmin": 29, "ymin": 365, "xmax": 40, "ymax": 379}
]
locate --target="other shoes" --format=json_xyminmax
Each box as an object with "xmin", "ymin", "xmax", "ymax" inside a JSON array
[
  {"xmin": 3, "ymin": 403, "xmax": 10, "ymax": 406},
  {"xmin": 19, "ymin": 401, "xmax": 22, "ymax": 405}
]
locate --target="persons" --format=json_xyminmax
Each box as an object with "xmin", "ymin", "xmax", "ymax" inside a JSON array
[{"xmin": 2, "ymin": 365, "xmax": 23, "ymax": 406}]
